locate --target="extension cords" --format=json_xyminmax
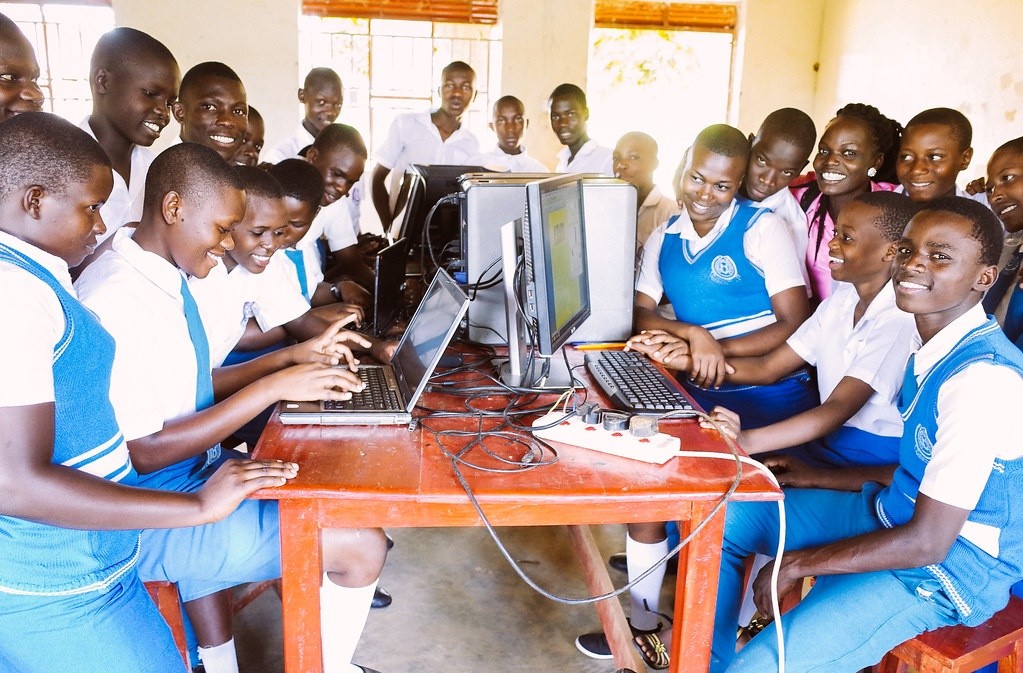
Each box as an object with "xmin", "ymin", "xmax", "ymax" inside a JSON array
[{"xmin": 532, "ymin": 413, "xmax": 682, "ymax": 464}]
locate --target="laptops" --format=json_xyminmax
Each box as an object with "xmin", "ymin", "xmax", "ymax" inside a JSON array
[
  {"xmin": 346, "ymin": 236, "xmax": 409, "ymax": 337},
  {"xmin": 280, "ymin": 267, "xmax": 471, "ymax": 424}
]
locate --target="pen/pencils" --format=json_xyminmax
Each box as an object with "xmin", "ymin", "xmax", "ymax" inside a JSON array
[{"xmin": 571, "ymin": 342, "xmax": 627, "ymax": 349}]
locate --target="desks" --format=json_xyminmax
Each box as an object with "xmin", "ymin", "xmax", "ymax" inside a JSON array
[{"xmin": 249, "ymin": 326, "xmax": 784, "ymax": 673}]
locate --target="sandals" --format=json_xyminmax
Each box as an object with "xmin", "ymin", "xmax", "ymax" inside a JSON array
[
  {"xmin": 746, "ymin": 617, "xmax": 770, "ymax": 640},
  {"xmin": 632, "ymin": 632, "xmax": 671, "ymax": 670}
]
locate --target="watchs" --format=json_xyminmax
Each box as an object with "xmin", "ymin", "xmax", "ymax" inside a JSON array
[{"xmin": 330, "ymin": 284, "xmax": 343, "ymax": 301}]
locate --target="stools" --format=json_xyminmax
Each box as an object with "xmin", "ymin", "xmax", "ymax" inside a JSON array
[{"xmin": 859, "ymin": 577, "xmax": 1023, "ymax": 673}]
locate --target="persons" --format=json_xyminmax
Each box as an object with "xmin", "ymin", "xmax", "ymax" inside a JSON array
[
  {"xmin": 612, "ymin": 131, "xmax": 679, "ymax": 274},
  {"xmin": 228, "ymin": 104, "xmax": 266, "ymax": 168},
  {"xmin": 896, "ymin": 107, "xmax": 1006, "ymax": 231},
  {"xmin": 788, "ymin": 103, "xmax": 904, "ymax": 311},
  {"xmin": 369, "ymin": 62, "xmax": 480, "ymax": 236},
  {"xmin": 0, "ymin": 111, "xmax": 301, "ymax": 671},
  {"xmin": 966, "ymin": 135, "xmax": 1023, "ymax": 350},
  {"xmin": 76, "ymin": 26, "xmax": 182, "ymax": 251},
  {"xmin": 263, "ymin": 66, "xmax": 363, "ymax": 237},
  {"xmin": 172, "ymin": 61, "xmax": 248, "ymax": 161},
  {"xmin": 547, "ymin": 84, "xmax": 615, "ymax": 175},
  {"xmin": 710, "ymin": 195, "xmax": 1023, "ymax": 673},
  {"xmin": 609, "ymin": 125, "xmax": 810, "ymax": 572},
  {"xmin": 672, "ymin": 107, "xmax": 817, "ymax": 298},
  {"xmin": 0, "ymin": 12, "xmax": 44, "ymax": 127},
  {"xmin": 578, "ymin": 189, "xmax": 921, "ymax": 657},
  {"xmin": 73, "ymin": 125, "xmax": 394, "ymax": 673},
  {"xmin": 481, "ymin": 95, "xmax": 550, "ymax": 173}
]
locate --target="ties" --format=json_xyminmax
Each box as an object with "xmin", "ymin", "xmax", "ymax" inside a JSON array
[
  {"xmin": 180, "ymin": 273, "xmax": 221, "ymax": 480},
  {"xmin": 902, "ymin": 353, "xmax": 917, "ymax": 407}
]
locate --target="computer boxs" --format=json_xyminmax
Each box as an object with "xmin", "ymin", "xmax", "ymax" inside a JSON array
[
  {"xmin": 416, "ymin": 165, "xmax": 487, "ymax": 252},
  {"xmin": 457, "ymin": 171, "xmax": 560, "ymax": 346}
]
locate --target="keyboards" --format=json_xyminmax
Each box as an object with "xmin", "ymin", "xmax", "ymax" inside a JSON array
[{"xmin": 583, "ymin": 351, "xmax": 697, "ymax": 417}]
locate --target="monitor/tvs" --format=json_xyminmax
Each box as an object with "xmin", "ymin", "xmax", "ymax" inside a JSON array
[
  {"xmin": 389, "ymin": 164, "xmax": 428, "ymax": 243},
  {"xmin": 501, "ymin": 175, "xmax": 638, "ymax": 395}
]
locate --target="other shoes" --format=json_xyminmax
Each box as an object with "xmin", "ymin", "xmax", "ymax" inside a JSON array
[
  {"xmin": 608, "ymin": 551, "xmax": 677, "ymax": 574},
  {"xmin": 384, "ymin": 533, "xmax": 394, "ymax": 549},
  {"xmin": 371, "ymin": 586, "xmax": 393, "ymax": 608},
  {"xmin": 354, "ymin": 663, "xmax": 380, "ymax": 673},
  {"xmin": 575, "ymin": 617, "xmax": 663, "ymax": 659}
]
